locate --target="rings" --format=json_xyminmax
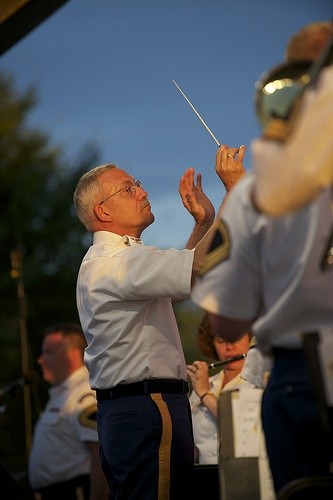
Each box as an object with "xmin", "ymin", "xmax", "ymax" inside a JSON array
[{"xmin": 227, "ymin": 154, "xmax": 233, "ymax": 158}]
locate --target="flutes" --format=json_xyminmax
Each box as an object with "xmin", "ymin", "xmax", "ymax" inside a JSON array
[{"xmin": 209, "ymin": 353, "xmax": 247, "ymax": 369}]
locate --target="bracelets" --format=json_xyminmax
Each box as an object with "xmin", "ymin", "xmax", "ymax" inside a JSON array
[{"xmin": 200, "ymin": 390, "xmax": 214, "ymax": 404}]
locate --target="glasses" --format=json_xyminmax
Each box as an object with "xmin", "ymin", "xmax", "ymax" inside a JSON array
[{"xmin": 99, "ymin": 180, "xmax": 141, "ymax": 206}]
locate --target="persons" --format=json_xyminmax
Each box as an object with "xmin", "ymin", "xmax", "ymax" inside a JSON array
[
  {"xmin": 73, "ymin": 144, "xmax": 246, "ymax": 500},
  {"xmin": 190, "ymin": 23, "xmax": 333, "ymax": 500},
  {"xmin": 186, "ymin": 309, "xmax": 277, "ymax": 500},
  {"xmin": 27, "ymin": 323, "xmax": 110, "ymax": 500}
]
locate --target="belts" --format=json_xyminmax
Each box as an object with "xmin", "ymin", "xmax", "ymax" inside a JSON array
[
  {"xmin": 96, "ymin": 379, "xmax": 190, "ymax": 399},
  {"xmin": 33, "ymin": 474, "xmax": 90, "ymax": 500}
]
